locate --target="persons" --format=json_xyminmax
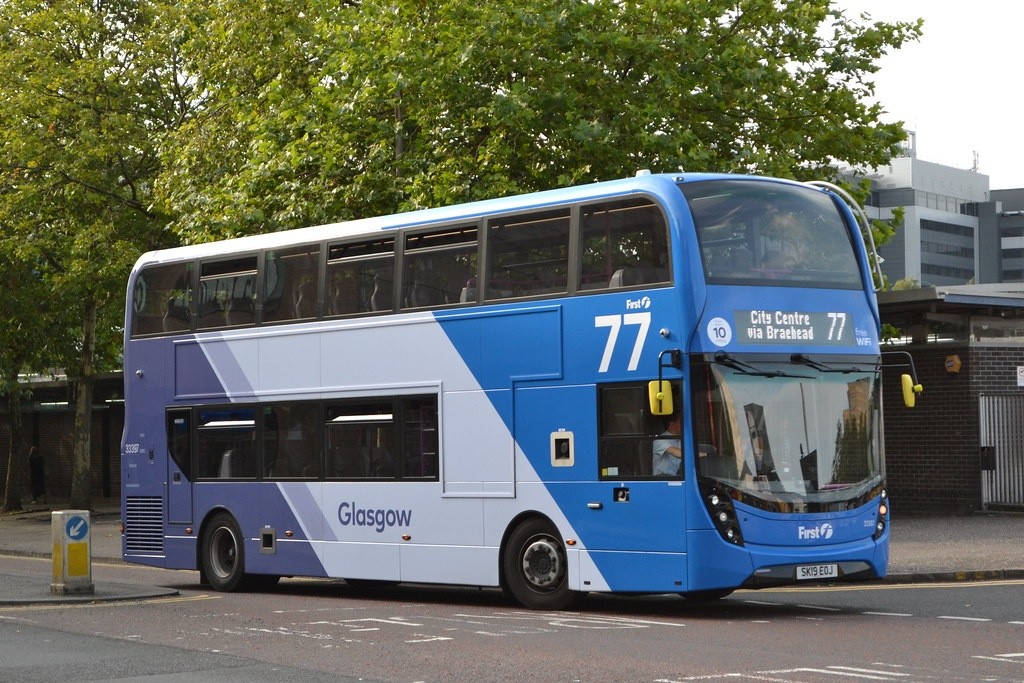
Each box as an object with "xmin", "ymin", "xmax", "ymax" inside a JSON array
[
  {"xmin": 28, "ymin": 446, "xmax": 47, "ymax": 505},
  {"xmin": 652, "ymin": 410, "xmax": 708, "ymax": 475}
]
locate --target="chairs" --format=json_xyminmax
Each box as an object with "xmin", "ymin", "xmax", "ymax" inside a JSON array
[
  {"xmin": 218, "ymin": 448, "xmax": 269, "ymax": 478},
  {"xmin": 638, "ymin": 409, "xmax": 664, "ymax": 479},
  {"xmin": 135, "ymin": 248, "xmax": 756, "ymax": 336}
]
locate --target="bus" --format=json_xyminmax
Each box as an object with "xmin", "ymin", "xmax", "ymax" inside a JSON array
[{"xmin": 120, "ymin": 168, "xmax": 924, "ymax": 610}]
